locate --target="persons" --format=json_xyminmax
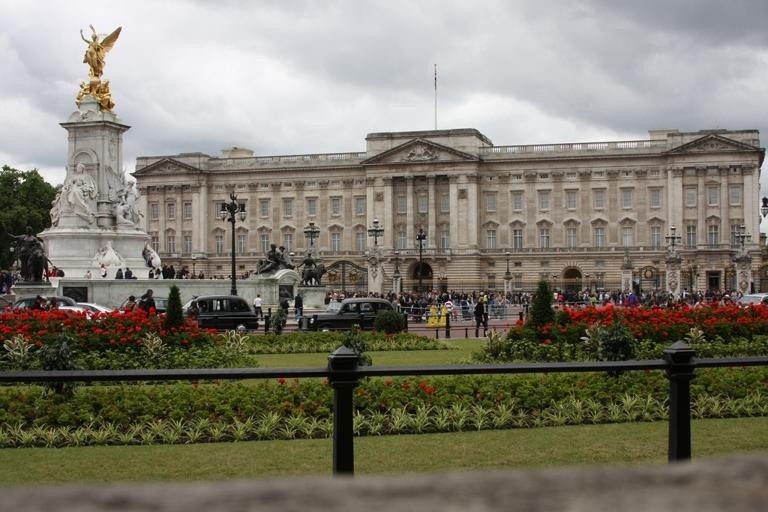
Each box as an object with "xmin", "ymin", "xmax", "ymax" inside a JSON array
[
  {"xmin": 275, "ymin": 244, "xmax": 296, "ymax": 269},
  {"xmin": 68, "ymin": 161, "xmax": 98, "ymax": 199},
  {"xmin": 5, "ymin": 226, "xmax": 40, "ymax": 254},
  {"xmin": 139, "ymin": 289, "xmax": 155, "ymax": 313},
  {"xmin": 325, "ymin": 289, "xmax": 533, "ymax": 324},
  {"xmin": 76, "ymin": 80, "xmax": 113, "ymax": 114},
  {"xmin": 124, "ymin": 267, "xmax": 132, "ymax": 278},
  {"xmin": 473, "ymin": 297, "xmax": 489, "ymax": 337},
  {"xmin": 552, "ymin": 288, "xmax": 743, "ymax": 308},
  {"xmin": 294, "ymin": 293, "xmax": 304, "ymax": 321},
  {"xmin": 296, "ymin": 253, "xmax": 318, "ymax": 285},
  {"xmin": 43, "ymin": 266, "xmax": 65, "ymax": 278},
  {"xmin": 115, "ymin": 268, "xmax": 123, "ymax": 279},
  {"xmin": 154, "ymin": 264, "xmax": 190, "ymax": 280},
  {"xmin": 148, "ymin": 269, "xmax": 154, "ymax": 279},
  {"xmin": 125, "ymin": 295, "xmax": 138, "ymax": 309},
  {"xmin": 253, "ymin": 243, "xmax": 284, "ymax": 273},
  {"xmin": 78, "ymin": 28, "xmax": 111, "ymax": 71},
  {"xmin": 83, "ymin": 270, "xmax": 92, "ymax": 279},
  {"xmin": 100, "ymin": 263, "xmax": 107, "ymax": 279},
  {"xmin": 251, "ymin": 294, "xmax": 265, "ymax": 321},
  {"xmin": 198, "ymin": 270, "xmax": 204, "ymax": 278},
  {"xmin": 118, "ymin": 180, "xmax": 145, "ymax": 219},
  {"xmin": 187, "ymin": 301, "xmax": 200, "ymax": 316},
  {"xmin": 28, "ymin": 294, "xmax": 58, "ymax": 312},
  {"xmin": 280, "ymin": 299, "xmax": 290, "ymax": 314}
]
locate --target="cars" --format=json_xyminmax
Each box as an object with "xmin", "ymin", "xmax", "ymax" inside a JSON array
[
  {"xmin": 734, "ymin": 292, "xmax": 768, "ymax": 306},
  {"xmin": 0, "ymin": 295, "xmax": 168, "ymax": 324},
  {"xmin": 156, "ymin": 294, "xmax": 259, "ymax": 334},
  {"xmin": 298, "ymin": 297, "xmax": 399, "ymax": 333}
]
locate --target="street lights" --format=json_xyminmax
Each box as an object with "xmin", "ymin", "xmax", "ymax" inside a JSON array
[
  {"xmin": 367, "ymin": 217, "xmax": 384, "ymax": 246},
  {"xmin": 218, "ymin": 190, "xmax": 248, "ymax": 296},
  {"xmin": 503, "ymin": 251, "xmax": 512, "ymax": 274},
  {"xmin": 303, "ymin": 220, "xmax": 320, "ymax": 246},
  {"xmin": 734, "ymin": 222, "xmax": 752, "ymax": 253},
  {"xmin": 416, "ymin": 228, "xmax": 426, "ymax": 290},
  {"xmin": 664, "ymin": 222, "xmax": 682, "ymax": 255},
  {"xmin": 393, "ymin": 249, "xmax": 399, "ymax": 273}
]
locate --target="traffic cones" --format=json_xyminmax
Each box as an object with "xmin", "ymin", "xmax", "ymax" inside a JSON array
[
  {"xmin": 425, "ymin": 304, "xmax": 439, "ymax": 328},
  {"xmin": 439, "ymin": 305, "xmax": 448, "ymax": 328}
]
